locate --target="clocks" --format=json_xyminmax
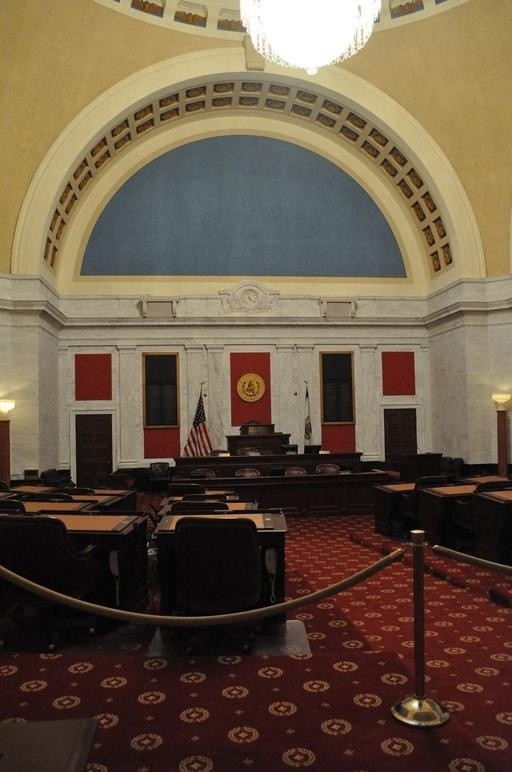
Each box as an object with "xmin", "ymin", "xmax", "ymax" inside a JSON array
[{"xmin": 238, "ymin": 284, "xmax": 263, "ymax": 313}]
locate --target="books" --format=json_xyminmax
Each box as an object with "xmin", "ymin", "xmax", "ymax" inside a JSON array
[
  {"xmin": 319, "ymin": 450, "xmax": 330, "ymax": 454},
  {"xmin": 212, "ymin": 448, "xmax": 230, "ymax": 457}
]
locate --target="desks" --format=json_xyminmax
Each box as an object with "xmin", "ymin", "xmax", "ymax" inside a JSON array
[{"xmin": 370, "ymin": 470, "xmax": 512, "ymax": 550}]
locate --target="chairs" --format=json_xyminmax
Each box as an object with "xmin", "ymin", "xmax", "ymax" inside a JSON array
[{"xmin": 285, "ymin": 463, "xmax": 348, "ymax": 517}]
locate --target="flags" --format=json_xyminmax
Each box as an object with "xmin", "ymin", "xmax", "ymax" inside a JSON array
[
  {"xmin": 301, "ymin": 387, "xmax": 313, "ymax": 440},
  {"xmin": 180, "ymin": 392, "xmax": 216, "ymax": 457}
]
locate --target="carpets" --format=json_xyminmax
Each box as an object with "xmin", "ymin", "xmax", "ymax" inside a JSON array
[{"xmin": 0, "ymin": 713, "xmax": 100, "ymax": 769}]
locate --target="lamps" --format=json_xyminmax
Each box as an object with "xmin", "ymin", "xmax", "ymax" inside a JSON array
[
  {"xmin": 0, "ymin": 400, "xmax": 15, "ymax": 481},
  {"xmin": 238, "ymin": 0, "xmax": 383, "ymax": 78},
  {"xmin": 490, "ymin": 392, "xmax": 512, "ymax": 474}
]
